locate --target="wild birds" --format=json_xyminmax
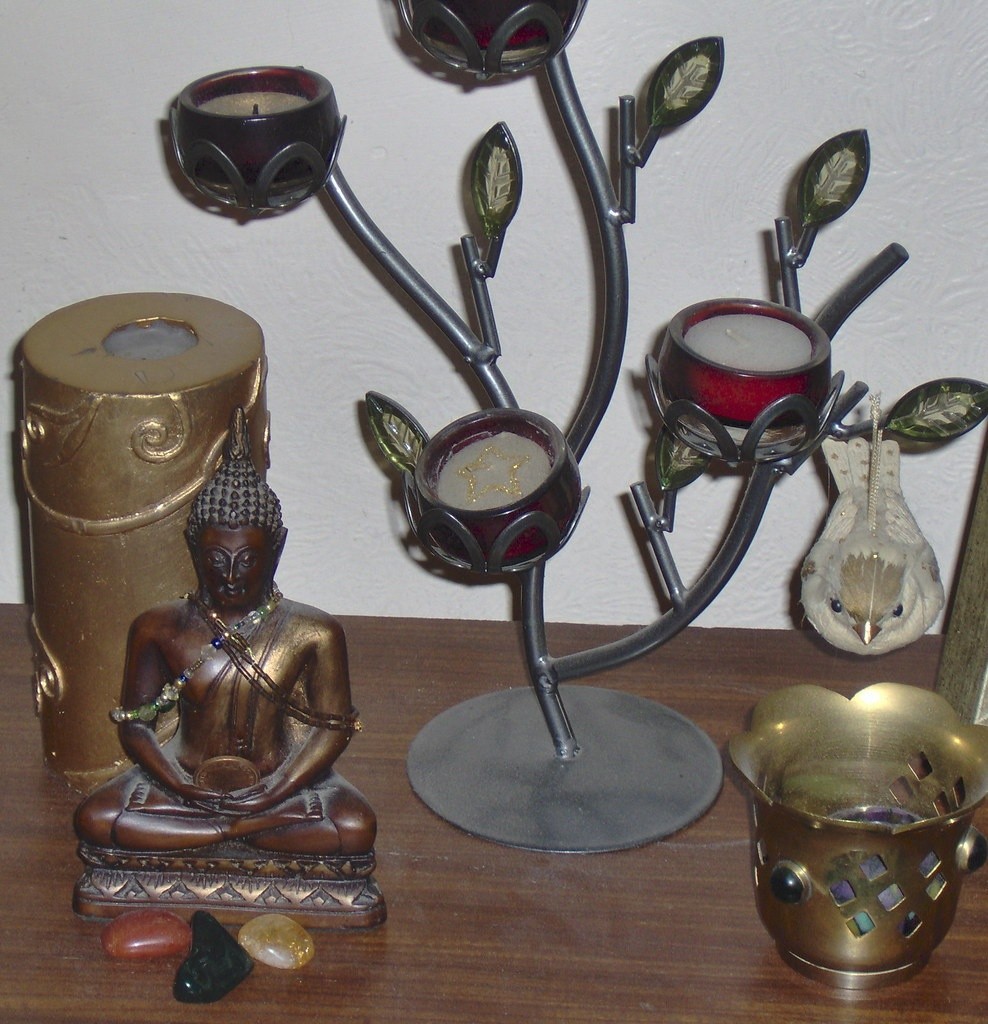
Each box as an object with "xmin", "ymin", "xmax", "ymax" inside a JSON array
[{"xmin": 797, "ymin": 395, "xmax": 945, "ymax": 656}]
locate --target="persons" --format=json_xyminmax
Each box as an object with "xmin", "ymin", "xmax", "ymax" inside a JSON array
[{"xmin": 75, "ymin": 406, "xmax": 375, "ymax": 855}]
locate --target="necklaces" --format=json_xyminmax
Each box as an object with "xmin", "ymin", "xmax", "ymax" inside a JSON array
[
  {"xmin": 108, "ymin": 581, "xmax": 283, "ymax": 724},
  {"xmin": 180, "ymin": 592, "xmax": 365, "ymax": 733}
]
